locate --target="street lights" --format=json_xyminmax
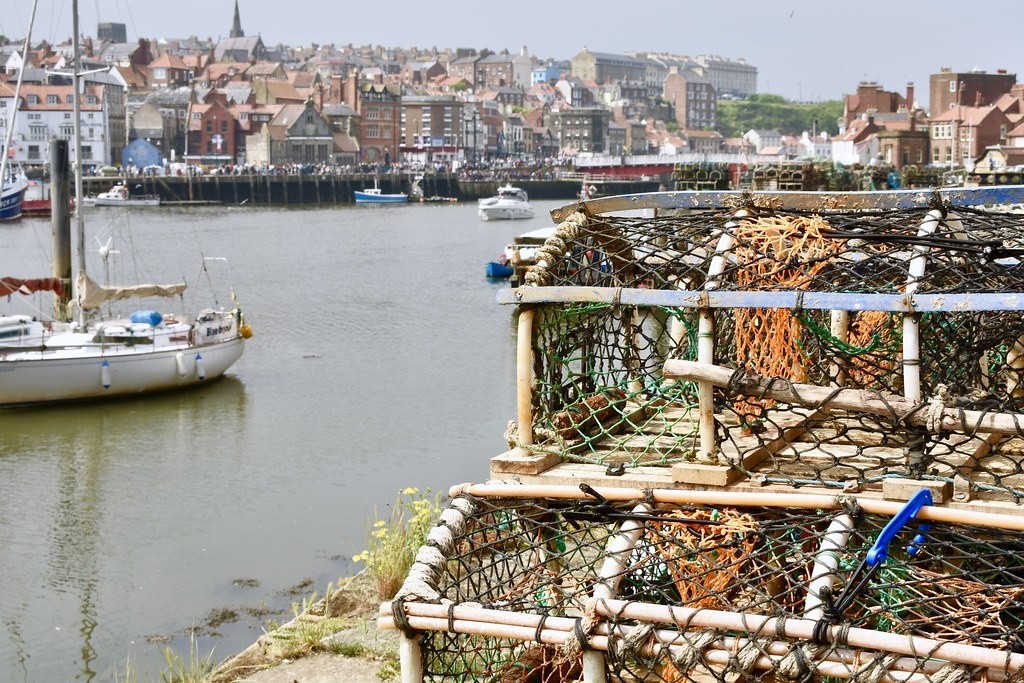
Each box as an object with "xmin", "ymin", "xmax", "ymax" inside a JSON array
[
  {"xmin": 472, "ymin": 110, "xmax": 479, "ymax": 168},
  {"xmin": 812, "ymin": 119, "xmax": 819, "ymax": 162}
]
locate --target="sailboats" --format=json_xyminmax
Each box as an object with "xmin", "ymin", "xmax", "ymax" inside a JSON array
[{"xmin": 0, "ymin": 0, "xmax": 255, "ymax": 406}]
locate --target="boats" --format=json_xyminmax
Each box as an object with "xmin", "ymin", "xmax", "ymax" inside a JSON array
[
  {"xmin": 488, "ymin": 227, "xmax": 605, "ymax": 288},
  {"xmin": 354, "ymin": 175, "xmax": 409, "ymax": 203},
  {"xmin": 477, "ymin": 182, "xmax": 535, "ymax": 222}
]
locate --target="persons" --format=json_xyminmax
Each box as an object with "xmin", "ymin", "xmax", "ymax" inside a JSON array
[{"xmin": 90, "ymin": 156, "xmax": 566, "ymax": 181}]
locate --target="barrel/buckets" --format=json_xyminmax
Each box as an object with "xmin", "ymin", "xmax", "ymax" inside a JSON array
[{"xmin": 131, "ymin": 311, "xmax": 163, "ymax": 326}]
